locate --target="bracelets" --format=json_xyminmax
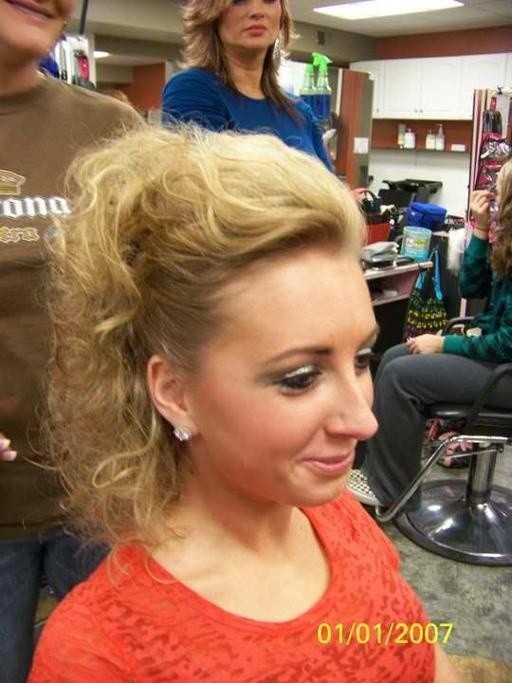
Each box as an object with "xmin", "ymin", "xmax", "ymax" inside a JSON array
[{"xmin": 474, "ymin": 225, "xmax": 490, "ymax": 231}]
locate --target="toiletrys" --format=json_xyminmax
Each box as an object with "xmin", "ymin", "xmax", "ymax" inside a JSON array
[
  {"xmin": 435, "ymin": 123, "xmax": 445, "ymax": 151},
  {"xmin": 404, "ymin": 128, "xmax": 416, "ymax": 149},
  {"xmin": 424, "ymin": 128, "xmax": 437, "ymax": 150}
]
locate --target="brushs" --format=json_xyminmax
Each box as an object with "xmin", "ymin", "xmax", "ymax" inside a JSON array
[{"xmin": 486, "ymin": 183, "xmax": 495, "ymax": 192}]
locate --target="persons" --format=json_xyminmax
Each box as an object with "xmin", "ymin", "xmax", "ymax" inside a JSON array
[
  {"xmin": 1, "ymin": 0, "xmax": 151, "ymax": 682},
  {"xmin": 160, "ymin": 0, "xmax": 336, "ymax": 175},
  {"xmin": 22, "ymin": 122, "xmax": 460, "ymax": 682},
  {"xmin": 338, "ymin": 157, "xmax": 511, "ymax": 514}
]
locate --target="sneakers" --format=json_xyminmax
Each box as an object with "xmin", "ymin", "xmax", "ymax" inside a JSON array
[{"xmin": 343, "ymin": 468, "xmax": 384, "ymax": 508}]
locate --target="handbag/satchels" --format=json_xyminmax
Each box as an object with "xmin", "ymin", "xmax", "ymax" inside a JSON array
[{"xmin": 400, "ymin": 247, "xmax": 450, "ymax": 344}]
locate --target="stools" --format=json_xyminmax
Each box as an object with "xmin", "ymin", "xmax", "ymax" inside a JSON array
[{"xmin": 374, "ymin": 312, "xmax": 512, "ymax": 567}]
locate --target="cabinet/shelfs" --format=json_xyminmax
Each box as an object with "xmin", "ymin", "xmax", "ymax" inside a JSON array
[{"xmin": 348, "ymin": 53, "xmax": 512, "ymax": 120}]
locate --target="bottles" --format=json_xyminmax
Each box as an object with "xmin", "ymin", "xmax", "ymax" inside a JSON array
[{"xmin": 299, "ymin": 63, "xmax": 316, "ymax": 116}]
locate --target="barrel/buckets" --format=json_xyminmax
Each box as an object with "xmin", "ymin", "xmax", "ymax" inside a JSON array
[
  {"xmin": 400, "ymin": 224, "xmax": 433, "ymax": 260},
  {"xmin": 407, "ymin": 200, "xmax": 447, "ymax": 233}
]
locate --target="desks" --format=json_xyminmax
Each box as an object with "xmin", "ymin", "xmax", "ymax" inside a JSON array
[{"xmin": 366, "ymin": 261, "xmax": 432, "ymax": 310}]
